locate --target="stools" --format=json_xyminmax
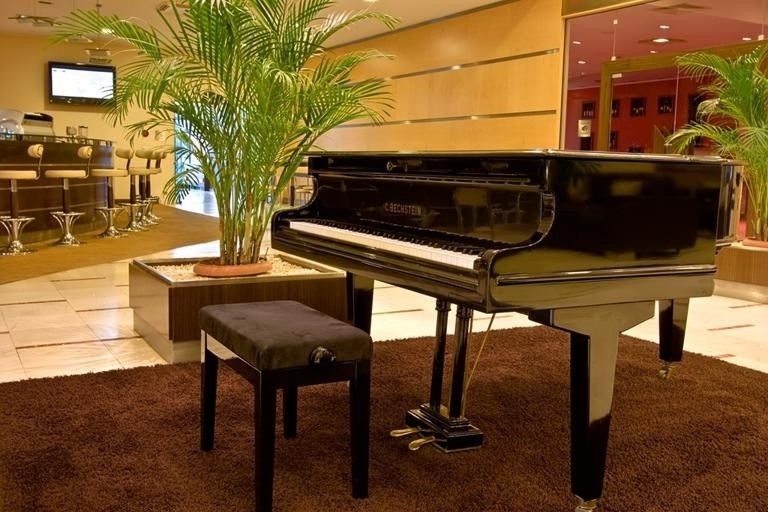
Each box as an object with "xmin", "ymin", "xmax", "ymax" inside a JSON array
[{"xmin": 195, "ymin": 301, "xmax": 374, "ymax": 509}]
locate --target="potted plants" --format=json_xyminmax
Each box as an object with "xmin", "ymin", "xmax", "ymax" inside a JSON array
[
  {"xmin": 43, "ymin": 0, "xmax": 403, "ymax": 362},
  {"xmin": 663, "ymin": 40, "xmax": 768, "ymax": 303}
]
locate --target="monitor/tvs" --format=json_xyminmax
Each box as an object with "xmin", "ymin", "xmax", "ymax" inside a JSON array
[{"xmin": 48, "ymin": 61, "xmax": 116, "ymax": 104}]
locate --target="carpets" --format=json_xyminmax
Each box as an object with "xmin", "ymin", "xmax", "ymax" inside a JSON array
[
  {"xmin": 0, "ymin": 319, "xmax": 765, "ymax": 509},
  {"xmin": 1, "ymin": 197, "xmax": 241, "ymax": 282}
]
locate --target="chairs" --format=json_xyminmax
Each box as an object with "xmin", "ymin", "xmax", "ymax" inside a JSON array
[{"xmin": 1, "ymin": 141, "xmax": 167, "ymax": 258}]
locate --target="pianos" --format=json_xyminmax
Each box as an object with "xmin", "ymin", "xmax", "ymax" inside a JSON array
[{"xmin": 270, "ymin": 148, "xmax": 751, "ymax": 512}]
